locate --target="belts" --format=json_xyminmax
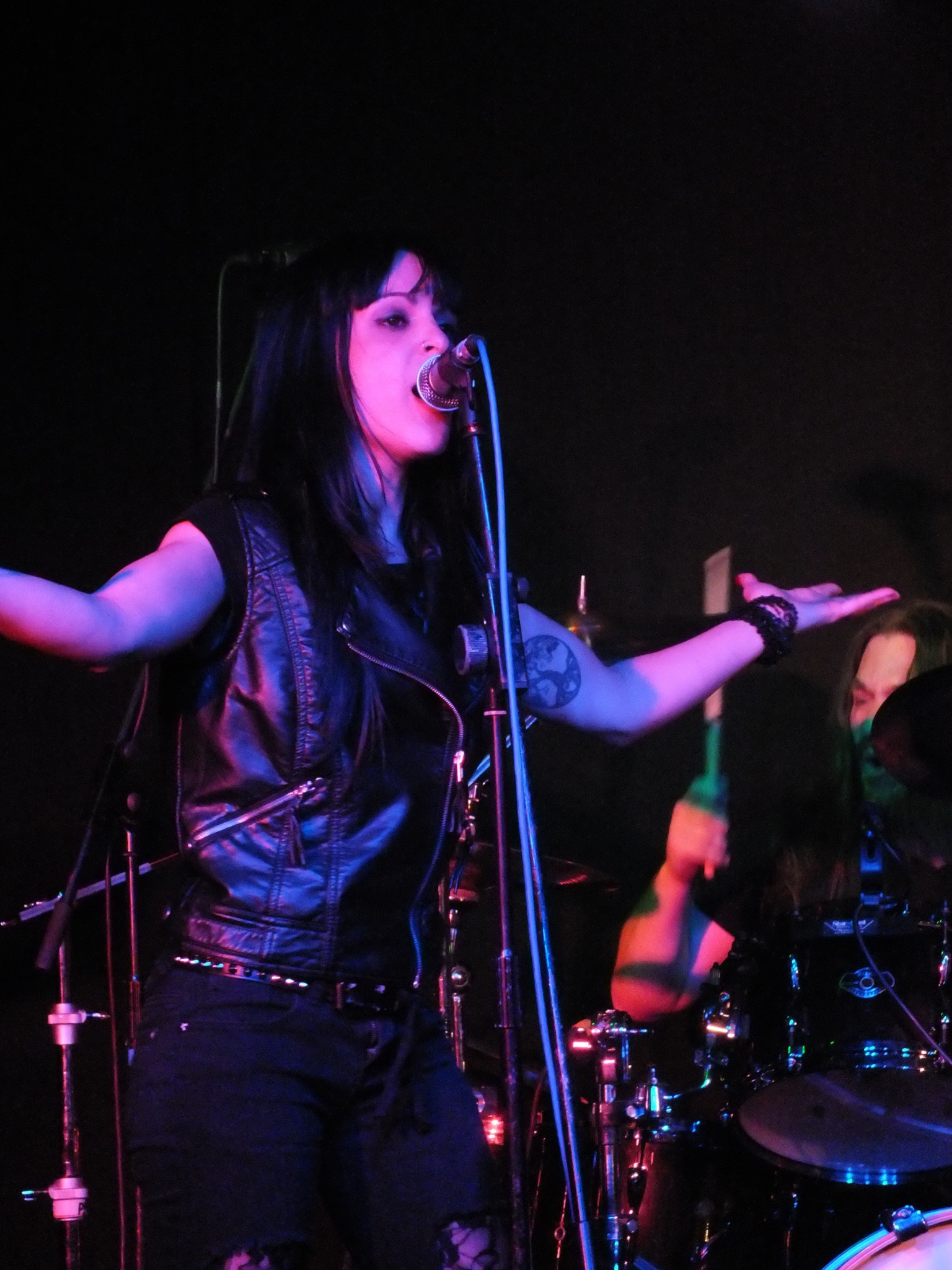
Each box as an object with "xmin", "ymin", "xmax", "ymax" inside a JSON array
[{"xmin": 171, "ymin": 948, "xmax": 406, "ymax": 1017}]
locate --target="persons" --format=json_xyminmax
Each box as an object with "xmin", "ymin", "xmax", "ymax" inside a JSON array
[
  {"xmin": 610, "ymin": 600, "xmax": 952, "ymax": 1268},
  {"xmin": 0, "ymin": 215, "xmax": 902, "ymax": 1266}
]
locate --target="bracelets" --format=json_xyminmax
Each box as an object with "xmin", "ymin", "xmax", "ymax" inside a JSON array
[{"xmin": 718, "ymin": 596, "xmax": 798, "ymax": 664}]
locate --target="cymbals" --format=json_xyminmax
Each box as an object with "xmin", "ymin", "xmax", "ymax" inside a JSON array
[
  {"xmin": 871, "ymin": 663, "xmax": 952, "ymax": 798},
  {"xmin": 435, "ymin": 840, "xmax": 625, "ymax": 906}
]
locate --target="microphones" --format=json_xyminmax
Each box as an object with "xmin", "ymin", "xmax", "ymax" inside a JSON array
[{"xmin": 417, "ymin": 330, "xmax": 479, "ymax": 414}]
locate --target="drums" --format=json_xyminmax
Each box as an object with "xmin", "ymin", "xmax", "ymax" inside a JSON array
[
  {"xmin": 556, "ymin": 1105, "xmax": 800, "ymax": 1269},
  {"xmin": 720, "ymin": 919, "xmax": 951, "ymax": 1189},
  {"xmin": 821, "ymin": 1207, "xmax": 951, "ymax": 1270}
]
